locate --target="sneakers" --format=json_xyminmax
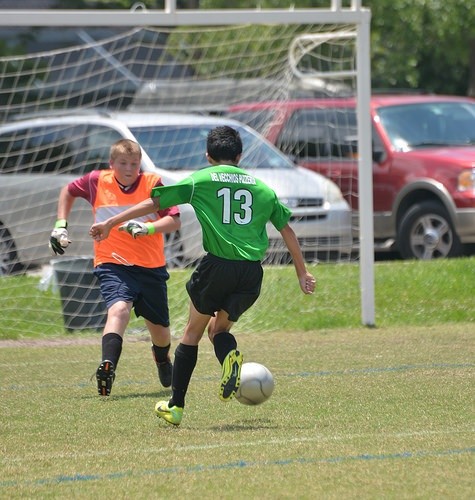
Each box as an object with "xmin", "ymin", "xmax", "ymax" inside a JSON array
[
  {"xmin": 90, "ymin": 359, "xmax": 116, "ymax": 397},
  {"xmin": 154, "ymin": 400, "xmax": 183, "ymax": 426},
  {"xmin": 151, "ymin": 344, "xmax": 172, "ymax": 387},
  {"xmin": 219, "ymin": 349, "xmax": 243, "ymax": 403}
]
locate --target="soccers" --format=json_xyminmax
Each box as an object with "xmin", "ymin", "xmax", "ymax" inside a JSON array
[{"xmin": 235, "ymin": 361, "xmax": 275, "ymax": 406}]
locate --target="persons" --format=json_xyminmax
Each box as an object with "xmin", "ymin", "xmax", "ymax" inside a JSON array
[
  {"xmin": 49, "ymin": 139, "xmax": 181, "ymax": 395},
  {"xmin": 89, "ymin": 126, "xmax": 315, "ymax": 426}
]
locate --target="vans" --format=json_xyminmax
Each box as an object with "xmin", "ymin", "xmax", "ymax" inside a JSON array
[
  {"xmin": 128, "ymin": 79, "xmax": 348, "ymax": 139},
  {"xmin": 226, "ymin": 96, "xmax": 475, "ymax": 261}
]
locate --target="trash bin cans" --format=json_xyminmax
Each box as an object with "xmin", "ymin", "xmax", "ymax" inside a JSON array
[{"xmin": 53, "ymin": 258, "xmax": 108, "ymax": 332}]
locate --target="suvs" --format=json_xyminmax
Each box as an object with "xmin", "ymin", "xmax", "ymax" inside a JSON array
[{"xmin": 0, "ymin": 107, "xmax": 352, "ymax": 274}]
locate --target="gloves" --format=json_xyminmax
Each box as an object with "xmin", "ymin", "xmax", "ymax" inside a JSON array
[
  {"xmin": 50, "ymin": 219, "xmax": 72, "ymax": 256},
  {"xmin": 118, "ymin": 220, "xmax": 154, "ymax": 239}
]
